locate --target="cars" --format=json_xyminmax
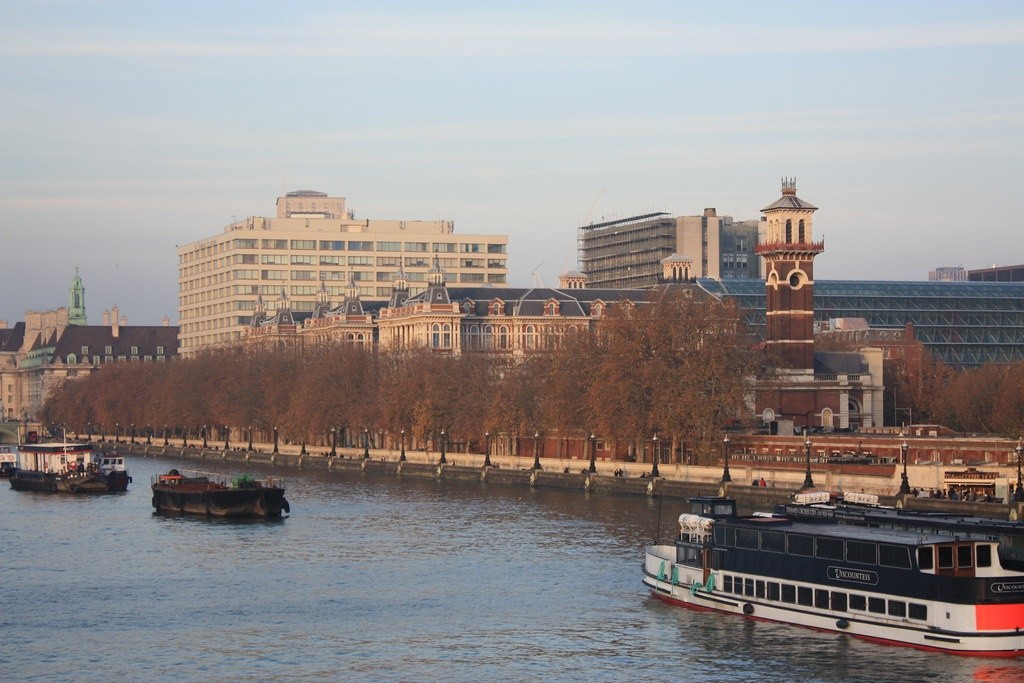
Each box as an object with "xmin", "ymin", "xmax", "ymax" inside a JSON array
[{"xmin": 821, "ymin": 447, "xmax": 1008, "ymax": 469}]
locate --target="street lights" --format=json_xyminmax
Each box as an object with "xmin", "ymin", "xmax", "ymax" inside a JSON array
[
  {"xmin": 1012, "ymin": 438, "xmax": 1024, "ymax": 500},
  {"xmin": 399, "ymin": 427, "xmax": 407, "ymax": 461},
  {"xmin": 651, "ymin": 433, "xmax": 660, "ymax": 476},
  {"xmin": 273, "ymin": 425, "xmax": 279, "ymax": 453},
  {"xmin": 330, "ymin": 426, "xmax": 337, "ymax": 457},
  {"xmin": 437, "ymin": 428, "xmax": 447, "ymax": 463},
  {"xmin": 899, "ymin": 440, "xmax": 911, "ymax": 495},
  {"xmin": 300, "ymin": 424, "xmax": 307, "ymax": 455},
  {"xmin": 800, "ymin": 436, "xmax": 816, "ymax": 490},
  {"xmin": 47, "ymin": 419, "xmax": 231, "ymax": 452},
  {"xmin": 721, "ymin": 434, "xmax": 730, "ymax": 482},
  {"xmin": 589, "ymin": 432, "xmax": 597, "ymax": 473},
  {"xmin": 363, "ymin": 428, "xmax": 370, "ymax": 458},
  {"xmin": 531, "ymin": 430, "xmax": 543, "ymax": 468},
  {"xmin": 248, "ymin": 425, "xmax": 253, "ymax": 451},
  {"xmin": 483, "ymin": 430, "xmax": 493, "ymax": 467}
]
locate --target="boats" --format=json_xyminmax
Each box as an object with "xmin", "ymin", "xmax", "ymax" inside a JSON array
[
  {"xmin": 0, "ymin": 445, "xmax": 17, "ymax": 476},
  {"xmin": 779, "ymin": 489, "xmax": 1024, "ymax": 571},
  {"xmin": 640, "ymin": 494, "xmax": 1024, "ymax": 661},
  {"xmin": 7, "ymin": 426, "xmax": 132, "ymax": 494},
  {"xmin": 150, "ymin": 466, "xmax": 291, "ymax": 523}
]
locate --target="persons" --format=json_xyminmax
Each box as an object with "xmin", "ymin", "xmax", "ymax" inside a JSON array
[
  {"xmin": 491, "ymin": 462, "xmax": 499, "ymax": 468},
  {"xmin": 615, "ymin": 469, "xmax": 624, "ymax": 477},
  {"xmin": 563, "ymin": 467, "xmax": 570, "ymax": 474},
  {"xmin": 911, "ymin": 487, "xmax": 992, "ymax": 503},
  {"xmin": 760, "ymin": 477, "xmax": 767, "ymax": 487},
  {"xmin": 640, "ymin": 471, "xmax": 652, "ymax": 478}
]
